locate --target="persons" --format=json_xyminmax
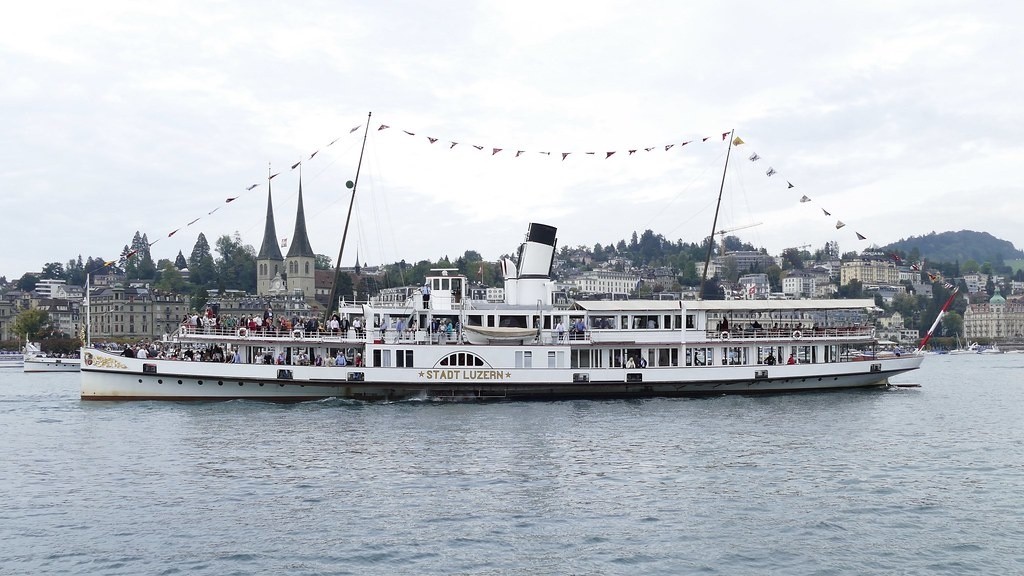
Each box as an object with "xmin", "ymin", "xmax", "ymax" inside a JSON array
[
  {"xmin": 626, "ymin": 357, "xmax": 635, "ymax": 369},
  {"xmin": 773, "ymin": 322, "xmax": 802, "ymax": 328},
  {"xmin": 764, "ymin": 353, "xmax": 775, "ymax": 365},
  {"xmin": 421, "ymin": 283, "xmax": 430, "ymax": 309},
  {"xmin": 732, "ymin": 324, "xmax": 743, "ymax": 330},
  {"xmin": 183, "ymin": 307, "xmax": 321, "ymax": 337},
  {"xmin": 326, "ymin": 316, "xmax": 361, "ymax": 339},
  {"xmin": 225, "ymin": 350, "xmax": 362, "ymax": 367},
  {"xmin": 380, "ymin": 318, "xmax": 453, "ymax": 343},
  {"xmin": 120, "ymin": 342, "xmax": 224, "ymax": 363},
  {"xmin": 787, "ymin": 354, "xmax": 795, "ymax": 364},
  {"xmin": 535, "ymin": 317, "xmax": 659, "ymax": 340},
  {"xmin": 715, "ymin": 314, "xmax": 729, "ymax": 332}
]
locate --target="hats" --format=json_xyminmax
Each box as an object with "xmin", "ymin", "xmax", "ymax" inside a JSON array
[
  {"xmin": 248, "ymin": 314, "xmax": 251, "ymax": 318},
  {"xmin": 242, "ymin": 315, "xmax": 245, "ymax": 318},
  {"xmin": 299, "ymin": 352, "xmax": 302, "ymax": 355}
]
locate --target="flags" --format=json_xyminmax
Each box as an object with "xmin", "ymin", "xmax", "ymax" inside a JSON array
[
  {"xmin": 732, "ymin": 136, "xmax": 777, "ymax": 177},
  {"xmin": 148, "ymin": 183, "xmax": 257, "ymax": 246},
  {"xmin": 749, "ymin": 286, "xmax": 755, "ymax": 295},
  {"xmin": 786, "ymin": 180, "xmax": 955, "ymax": 290},
  {"xmin": 103, "ymin": 250, "xmax": 138, "ymax": 267},
  {"xmin": 289, "ymin": 121, "xmax": 730, "ymax": 170},
  {"xmin": 267, "ymin": 173, "xmax": 278, "ymax": 180}
]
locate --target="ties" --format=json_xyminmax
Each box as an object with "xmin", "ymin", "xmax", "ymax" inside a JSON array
[{"xmin": 427, "ymin": 287, "xmax": 429, "ymax": 295}]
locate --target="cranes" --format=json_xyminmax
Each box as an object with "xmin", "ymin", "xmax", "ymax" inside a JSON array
[{"xmin": 708, "ymin": 221, "xmax": 763, "ymax": 256}]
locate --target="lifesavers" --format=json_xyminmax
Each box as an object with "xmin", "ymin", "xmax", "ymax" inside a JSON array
[
  {"xmin": 720, "ymin": 331, "xmax": 730, "ymax": 340},
  {"xmin": 792, "ymin": 330, "xmax": 801, "ymax": 340},
  {"xmin": 238, "ymin": 327, "xmax": 249, "ymax": 338},
  {"xmin": 180, "ymin": 326, "xmax": 186, "ymax": 336},
  {"xmin": 871, "ymin": 329, "xmax": 876, "ymax": 337},
  {"xmin": 292, "ymin": 329, "xmax": 303, "ymax": 341}
]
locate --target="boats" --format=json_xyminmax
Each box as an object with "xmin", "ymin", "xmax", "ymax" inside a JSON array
[
  {"xmin": 921, "ymin": 348, "xmax": 949, "ymax": 355},
  {"xmin": 23, "ymin": 332, "xmax": 124, "ymax": 372},
  {"xmin": 79, "ymin": 110, "xmax": 960, "ymax": 401},
  {"xmin": 976, "ymin": 341, "xmax": 1002, "ymax": 355},
  {"xmin": 947, "ymin": 331, "xmax": 980, "ymax": 356},
  {"xmin": 1003, "ymin": 348, "xmax": 1024, "ymax": 354}
]
